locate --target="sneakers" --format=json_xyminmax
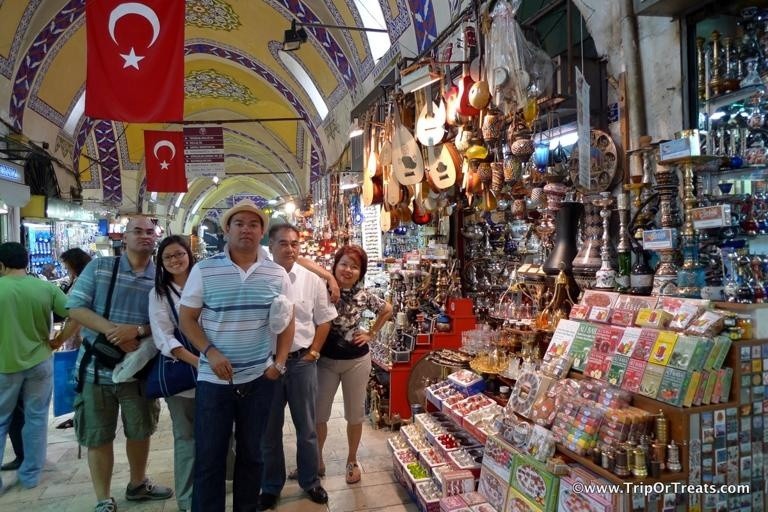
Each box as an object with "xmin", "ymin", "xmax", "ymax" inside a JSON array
[
  {"xmin": 95, "ymin": 497, "xmax": 117, "ymax": 512},
  {"xmin": 125, "ymin": 478, "xmax": 173, "ymax": 500}
]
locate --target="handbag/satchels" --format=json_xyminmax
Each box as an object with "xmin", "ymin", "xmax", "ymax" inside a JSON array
[
  {"xmin": 145, "ymin": 285, "xmax": 199, "ymax": 399},
  {"xmin": 73, "ymin": 256, "xmax": 150, "ymax": 392}
]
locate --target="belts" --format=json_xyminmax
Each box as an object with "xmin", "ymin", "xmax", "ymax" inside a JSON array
[{"xmin": 287, "ymin": 345, "xmax": 311, "ymax": 360}]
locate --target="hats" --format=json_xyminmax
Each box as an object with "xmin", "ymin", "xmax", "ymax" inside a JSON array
[{"xmin": 219, "ymin": 199, "xmax": 269, "ymax": 233}]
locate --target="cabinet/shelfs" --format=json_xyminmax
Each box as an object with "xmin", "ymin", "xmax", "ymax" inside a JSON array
[
  {"xmin": 422, "ymin": 292, "xmax": 767, "ymax": 512},
  {"xmin": 366, "ymin": 295, "xmax": 476, "ymax": 432}
]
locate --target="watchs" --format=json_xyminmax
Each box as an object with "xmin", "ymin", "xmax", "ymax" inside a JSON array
[
  {"xmin": 309, "ymin": 351, "xmax": 321, "ymax": 360},
  {"xmin": 137, "ymin": 324, "xmax": 145, "ymax": 338},
  {"xmin": 272, "ymin": 362, "xmax": 286, "ymax": 374}
]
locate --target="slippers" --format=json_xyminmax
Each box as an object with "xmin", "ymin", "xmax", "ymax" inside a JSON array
[
  {"xmin": 55, "ymin": 419, "xmax": 73, "ymax": 429},
  {"xmin": 346, "ymin": 463, "xmax": 361, "ymax": 483},
  {"xmin": 288, "ymin": 468, "xmax": 325, "ymax": 480}
]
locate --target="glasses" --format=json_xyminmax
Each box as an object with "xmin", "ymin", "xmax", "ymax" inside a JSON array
[
  {"xmin": 229, "ymin": 367, "xmax": 256, "ymax": 399},
  {"xmin": 127, "ymin": 229, "xmax": 156, "ymax": 237},
  {"xmin": 162, "ymin": 251, "xmax": 188, "ymax": 261}
]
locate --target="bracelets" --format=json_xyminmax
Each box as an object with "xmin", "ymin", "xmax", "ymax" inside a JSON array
[
  {"xmin": 203, "ymin": 343, "xmax": 214, "ymax": 357},
  {"xmin": 370, "ymin": 327, "xmax": 378, "ymax": 336}
]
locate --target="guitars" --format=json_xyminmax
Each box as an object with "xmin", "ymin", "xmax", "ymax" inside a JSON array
[
  {"xmin": 455, "ymin": 26, "xmax": 480, "ymax": 116},
  {"xmin": 411, "ymin": 199, "xmax": 431, "ymax": 226},
  {"xmin": 442, "ymin": 43, "xmax": 459, "ymax": 126}
]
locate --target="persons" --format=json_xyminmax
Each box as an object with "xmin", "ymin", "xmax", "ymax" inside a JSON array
[
  {"xmin": 178, "ymin": 198, "xmax": 296, "ymax": 511},
  {"xmin": 3, "ymin": 384, "xmax": 25, "ymax": 472},
  {"xmin": 148, "ymin": 236, "xmax": 201, "ymax": 512},
  {"xmin": 64, "ymin": 216, "xmax": 172, "ymax": 511},
  {"xmin": 288, "ymin": 246, "xmax": 393, "ymax": 483},
  {"xmin": 0, "ymin": 242, "xmax": 82, "ymax": 494},
  {"xmin": 256, "ymin": 225, "xmax": 337, "ymax": 510},
  {"xmin": 61, "ymin": 248, "xmax": 92, "ymax": 277}
]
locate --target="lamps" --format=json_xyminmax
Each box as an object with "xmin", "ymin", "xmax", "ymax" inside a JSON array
[
  {"xmin": 285, "ymin": 202, "xmax": 295, "ymax": 213},
  {"xmin": 283, "ymin": 18, "xmax": 389, "ymax": 53}
]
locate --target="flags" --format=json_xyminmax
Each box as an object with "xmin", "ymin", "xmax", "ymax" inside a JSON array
[
  {"xmin": 145, "ymin": 130, "xmax": 187, "ymax": 193},
  {"xmin": 85, "ymin": 0, "xmax": 186, "ymax": 122}
]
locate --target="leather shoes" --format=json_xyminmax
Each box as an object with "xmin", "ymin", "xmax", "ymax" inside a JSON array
[
  {"xmin": 257, "ymin": 493, "xmax": 276, "ymax": 511},
  {"xmin": 2, "ymin": 457, "xmax": 24, "ymax": 470},
  {"xmin": 308, "ymin": 487, "xmax": 328, "ymax": 504}
]
